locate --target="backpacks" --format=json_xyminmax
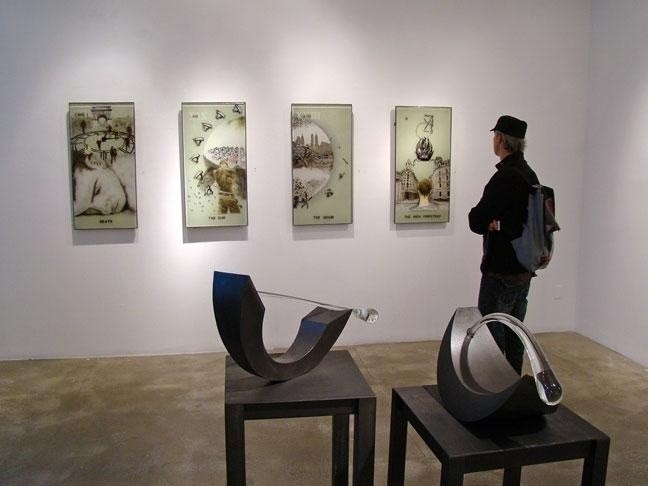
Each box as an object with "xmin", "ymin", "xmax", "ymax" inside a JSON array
[{"xmin": 511, "ymin": 166, "xmax": 560, "ymax": 275}]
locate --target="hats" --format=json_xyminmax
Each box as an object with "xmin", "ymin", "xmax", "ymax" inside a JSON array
[{"xmin": 490, "ymin": 115, "xmax": 527, "ymax": 138}]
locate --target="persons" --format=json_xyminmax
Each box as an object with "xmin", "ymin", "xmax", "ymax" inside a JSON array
[
  {"xmin": 213, "ymin": 160, "xmax": 242, "ymax": 213},
  {"xmin": 79, "ymin": 117, "xmax": 133, "ymax": 165},
  {"xmin": 416, "ymin": 179, "xmax": 435, "ymax": 207},
  {"xmin": 467, "ymin": 115, "xmax": 546, "ymax": 381},
  {"xmin": 71, "ymin": 146, "xmax": 137, "ymax": 218}
]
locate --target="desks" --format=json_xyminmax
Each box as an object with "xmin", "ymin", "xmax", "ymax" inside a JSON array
[
  {"xmin": 386, "ymin": 384, "xmax": 611, "ymax": 486},
  {"xmin": 224, "ymin": 349, "xmax": 379, "ymax": 486}
]
